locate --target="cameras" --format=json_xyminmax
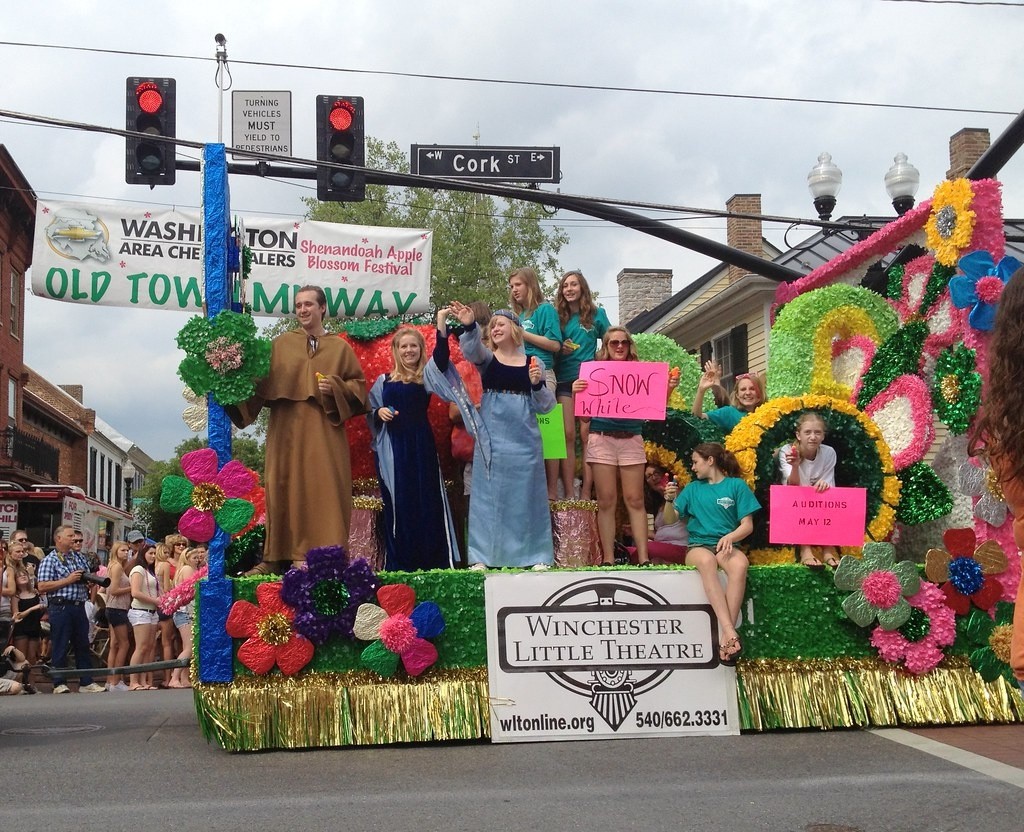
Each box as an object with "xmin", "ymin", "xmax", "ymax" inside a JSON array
[{"xmin": 79, "ymin": 570, "xmax": 111, "ymax": 588}]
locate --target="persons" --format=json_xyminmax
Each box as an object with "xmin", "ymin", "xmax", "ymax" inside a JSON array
[
  {"xmin": 967, "ymin": 266, "xmax": 1023, "ymax": 692},
  {"xmin": 704, "ymin": 360, "xmax": 768, "ymax": 408},
  {"xmin": 366, "ymin": 309, "xmax": 460, "ymax": 572},
  {"xmin": 509, "ymin": 267, "xmax": 562, "ymax": 500},
  {"xmin": 0, "ymin": 524, "xmax": 210, "ymax": 695},
  {"xmin": 572, "ymin": 327, "xmax": 682, "ymax": 568},
  {"xmin": 622, "ymin": 463, "xmax": 688, "ymax": 565},
  {"xmin": 224, "ymin": 285, "xmax": 373, "ymax": 576},
  {"xmin": 692, "ymin": 370, "xmax": 764, "ymax": 433},
  {"xmin": 448, "ymin": 320, "xmax": 493, "ymax": 515},
  {"xmin": 779, "ymin": 414, "xmax": 840, "ymax": 566},
  {"xmin": 450, "ymin": 300, "xmax": 557, "ymax": 570},
  {"xmin": 554, "ymin": 271, "xmax": 611, "ymax": 499},
  {"xmin": 663, "ymin": 443, "xmax": 762, "ymax": 667}
]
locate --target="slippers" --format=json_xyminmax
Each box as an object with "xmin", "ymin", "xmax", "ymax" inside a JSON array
[
  {"xmin": 718, "ymin": 633, "xmax": 743, "ymax": 666},
  {"xmin": 128, "ymin": 682, "xmax": 157, "ymax": 691},
  {"xmin": 799, "ymin": 556, "xmax": 825, "ymax": 569},
  {"xmin": 823, "ymin": 554, "xmax": 840, "ymax": 568}
]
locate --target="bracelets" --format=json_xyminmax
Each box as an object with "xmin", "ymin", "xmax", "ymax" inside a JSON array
[{"xmin": 666, "ymin": 500, "xmax": 673, "ymax": 503}]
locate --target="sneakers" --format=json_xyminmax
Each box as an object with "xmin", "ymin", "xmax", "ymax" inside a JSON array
[
  {"xmin": 105, "ymin": 682, "xmax": 128, "ymax": 691},
  {"xmin": 78, "ymin": 684, "xmax": 105, "ymax": 693},
  {"xmin": 53, "ymin": 685, "xmax": 70, "ymax": 693}
]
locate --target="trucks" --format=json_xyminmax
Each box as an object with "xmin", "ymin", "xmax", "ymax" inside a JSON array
[{"xmin": 0, "ymin": 480, "xmax": 133, "ymax": 566}]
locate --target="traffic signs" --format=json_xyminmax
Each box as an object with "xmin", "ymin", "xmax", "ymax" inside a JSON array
[{"xmin": 411, "ymin": 145, "xmax": 560, "ymax": 184}]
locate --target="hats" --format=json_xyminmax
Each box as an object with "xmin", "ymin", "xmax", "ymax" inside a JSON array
[{"xmin": 128, "ymin": 530, "xmax": 144, "ymax": 543}]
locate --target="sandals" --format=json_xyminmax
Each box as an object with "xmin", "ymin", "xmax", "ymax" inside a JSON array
[{"xmin": 243, "ymin": 566, "xmax": 280, "ymax": 579}]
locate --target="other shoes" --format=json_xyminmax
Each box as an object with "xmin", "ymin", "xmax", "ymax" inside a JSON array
[
  {"xmin": 599, "ymin": 561, "xmax": 609, "ymax": 567},
  {"xmin": 470, "ymin": 561, "xmax": 486, "ymax": 571},
  {"xmin": 641, "ymin": 560, "xmax": 651, "ymax": 567},
  {"xmin": 534, "ymin": 561, "xmax": 548, "ymax": 572}
]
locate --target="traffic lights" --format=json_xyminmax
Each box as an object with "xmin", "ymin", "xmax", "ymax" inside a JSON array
[
  {"xmin": 127, "ymin": 78, "xmax": 176, "ymax": 190},
  {"xmin": 316, "ymin": 95, "xmax": 366, "ymax": 202}
]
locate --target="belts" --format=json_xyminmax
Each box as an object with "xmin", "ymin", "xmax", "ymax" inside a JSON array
[
  {"xmin": 131, "ymin": 607, "xmax": 155, "ymax": 614},
  {"xmin": 63, "ymin": 599, "xmax": 84, "ymax": 605}
]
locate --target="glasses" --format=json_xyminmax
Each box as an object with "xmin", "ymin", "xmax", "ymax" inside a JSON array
[
  {"xmin": 74, "ymin": 538, "xmax": 83, "ymax": 544},
  {"xmin": 16, "ymin": 538, "xmax": 27, "ymax": 542},
  {"xmin": 132, "ymin": 539, "xmax": 143, "ymax": 545},
  {"xmin": 174, "ymin": 543, "xmax": 186, "ymax": 546},
  {"xmin": 735, "ymin": 371, "xmax": 756, "ymax": 379},
  {"xmin": 646, "ymin": 468, "xmax": 663, "ymax": 482},
  {"xmin": 606, "ymin": 340, "xmax": 631, "ymax": 349}
]
